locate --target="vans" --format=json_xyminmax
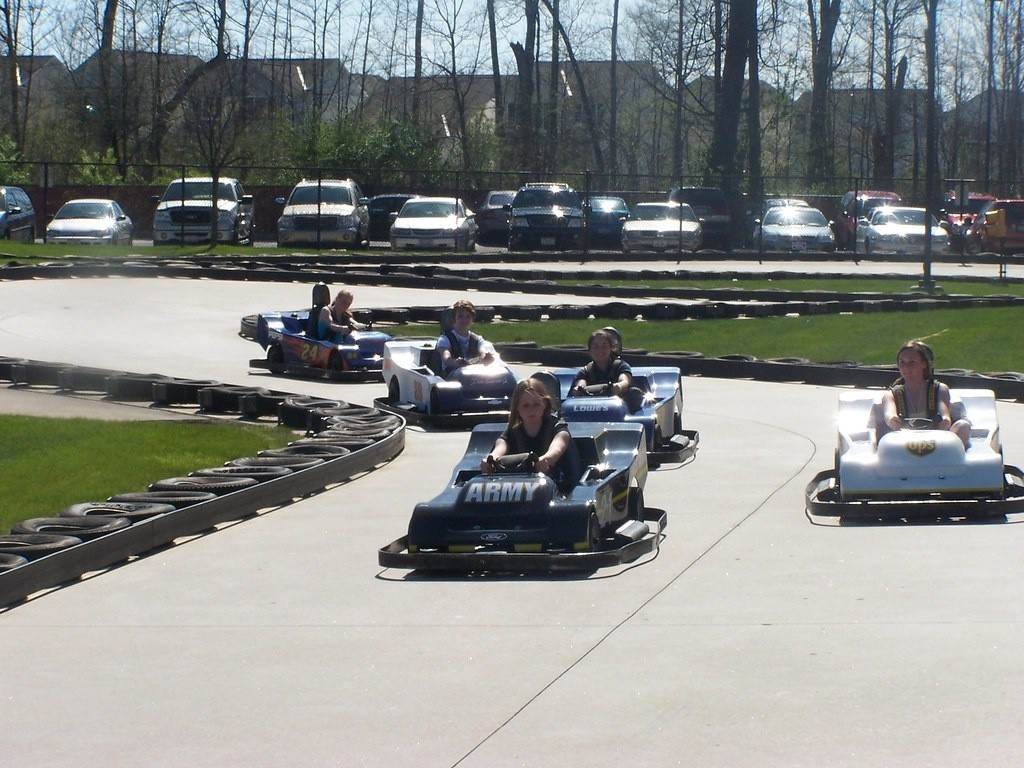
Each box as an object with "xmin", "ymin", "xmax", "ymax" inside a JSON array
[{"xmin": 964, "ymin": 199, "xmax": 1024, "ymax": 257}]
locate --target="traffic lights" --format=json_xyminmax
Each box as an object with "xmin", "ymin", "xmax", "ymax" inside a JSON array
[{"xmin": 985, "ymin": 208, "xmax": 1007, "ymax": 239}]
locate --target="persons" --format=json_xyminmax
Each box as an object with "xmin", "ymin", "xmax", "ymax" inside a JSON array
[
  {"xmin": 437, "ymin": 299, "xmax": 495, "ymax": 370},
  {"xmin": 479, "ymin": 377, "xmax": 573, "ymax": 496},
  {"xmin": 881, "ymin": 340, "xmax": 971, "ymax": 449},
  {"xmin": 317, "ymin": 290, "xmax": 368, "ymax": 344},
  {"xmin": 572, "ymin": 330, "xmax": 643, "ymax": 414}
]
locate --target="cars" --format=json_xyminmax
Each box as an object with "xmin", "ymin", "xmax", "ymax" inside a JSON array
[
  {"xmin": 752, "ymin": 206, "xmax": 836, "ymax": 261},
  {"xmin": 472, "ymin": 191, "xmax": 518, "ymax": 244},
  {"xmin": 389, "ymin": 196, "xmax": 479, "ymax": 251},
  {"xmin": 760, "ymin": 197, "xmax": 810, "ymax": 218},
  {"xmin": 618, "ymin": 202, "xmax": 706, "ymax": 254},
  {"xmin": 45, "ymin": 198, "xmax": 133, "ymax": 247},
  {"xmin": 858, "ymin": 206, "xmax": 951, "ymax": 255}
]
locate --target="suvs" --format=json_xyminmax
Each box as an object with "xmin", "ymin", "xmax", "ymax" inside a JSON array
[
  {"xmin": 934, "ymin": 191, "xmax": 998, "ymax": 252},
  {"xmin": 835, "ymin": 190, "xmax": 903, "ymax": 252},
  {"xmin": 582, "ymin": 195, "xmax": 633, "ymax": 248},
  {"xmin": 502, "ymin": 181, "xmax": 588, "ymax": 251},
  {"xmin": 150, "ymin": 176, "xmax": 255, "ymax": 248},
  {"xmin": 368, "ymin": 193, "xmax": 418, "ymax": 242},
  {"xmin": 275, "ymin": 177, "xmax": 371, "ymax": 251},
  {"xmin": 670, "ymin": 186, "xmax": 734, "ymax": 254},
  {"xmin": 0, "ymin": 186, "xmax": 36, "ymax": 243}
]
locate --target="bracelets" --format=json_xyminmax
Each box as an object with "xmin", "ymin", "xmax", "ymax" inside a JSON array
[{"xmin": 615, "ymin": 383, "xmax": 621, "ymax": 393}]
